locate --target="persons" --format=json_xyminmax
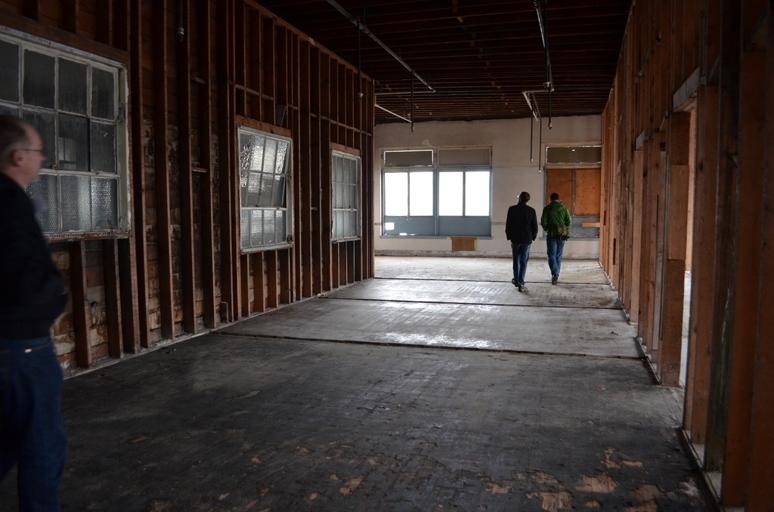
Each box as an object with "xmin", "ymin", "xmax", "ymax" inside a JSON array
[
  {"xmin": 541, "ymin": 192, "xmax": 571, "ymax": 285},
  {"xmin": 504, "ymin": 191, "xmax": 537, "ymax": 293},
  {"xmin": 0, "ymin": 115, "xmax": 72, "ymax": 512}
]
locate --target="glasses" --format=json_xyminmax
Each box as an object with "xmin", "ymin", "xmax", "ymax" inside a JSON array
[{"xmin": 18, "ymin": 147, "xmax": 49, "ymax": 157}]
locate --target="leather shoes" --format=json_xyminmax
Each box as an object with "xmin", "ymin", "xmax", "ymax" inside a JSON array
[
  {"xmin": 511, "ymin": 279, "xmax": 527, "ymax": 291},
  {"xmin": 551, "ymin": 274, "xmax": 559, "ymax": 285}
]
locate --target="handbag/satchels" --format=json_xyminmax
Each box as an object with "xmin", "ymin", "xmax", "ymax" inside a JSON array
[{"xmin": 555, "ymin": 224, "xmax": 571, "ymax": 239}]
